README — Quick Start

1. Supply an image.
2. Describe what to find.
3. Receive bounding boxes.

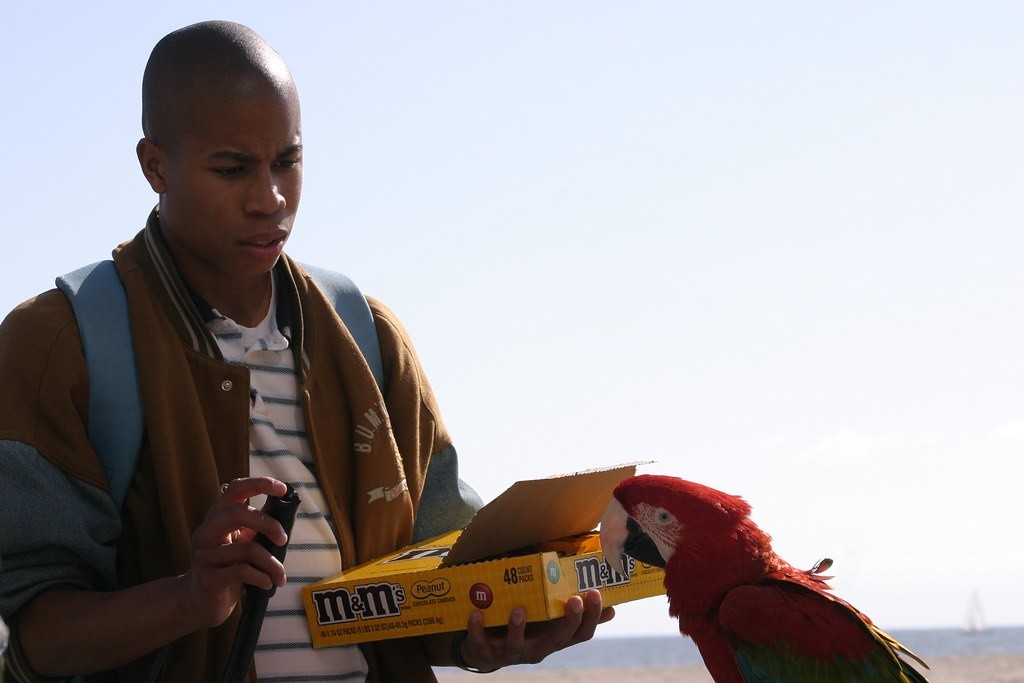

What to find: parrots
[599,474,932,683]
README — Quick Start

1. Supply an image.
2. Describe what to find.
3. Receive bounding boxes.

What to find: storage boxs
[299,458,667,651]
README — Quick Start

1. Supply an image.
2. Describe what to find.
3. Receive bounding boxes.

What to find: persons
[0,19,616,683]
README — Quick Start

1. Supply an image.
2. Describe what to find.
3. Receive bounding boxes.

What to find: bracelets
[450,630,502,673]
